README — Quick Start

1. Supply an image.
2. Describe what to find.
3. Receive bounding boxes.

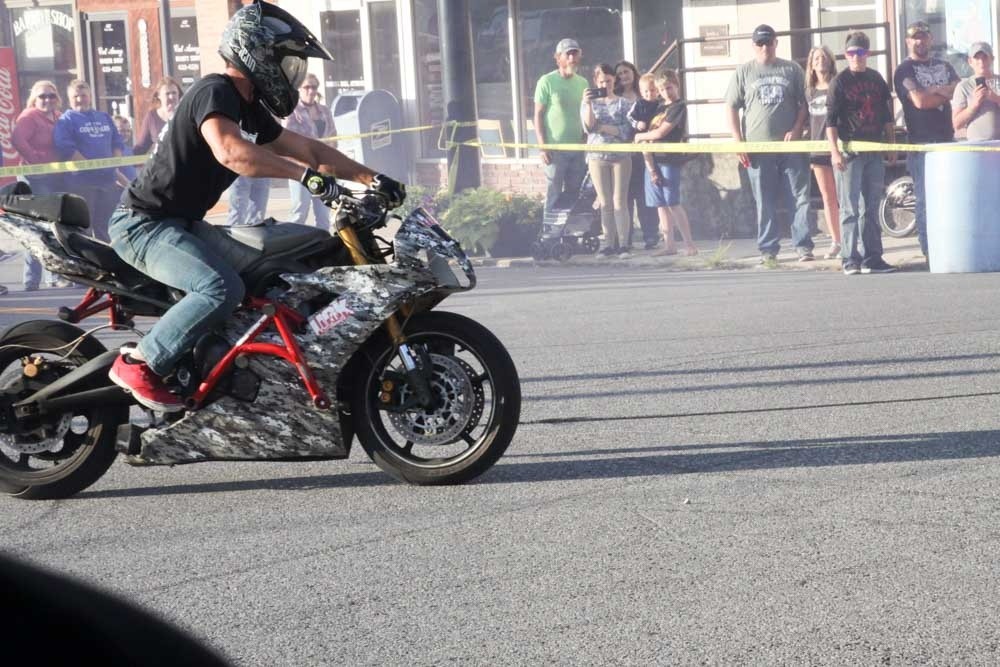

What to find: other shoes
[823,242,842,258]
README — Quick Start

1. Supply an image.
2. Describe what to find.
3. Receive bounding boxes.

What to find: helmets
[217,0,335,120]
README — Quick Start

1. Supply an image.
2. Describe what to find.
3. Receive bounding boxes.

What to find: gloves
[300,167,339,205]
[371,174,407,208]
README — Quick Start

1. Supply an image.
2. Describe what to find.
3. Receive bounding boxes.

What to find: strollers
[532,169,604,262]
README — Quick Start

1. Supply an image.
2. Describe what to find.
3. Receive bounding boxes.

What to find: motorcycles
[0,177,521,499]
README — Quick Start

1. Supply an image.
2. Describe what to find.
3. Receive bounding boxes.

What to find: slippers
[651,247,677,256]
[686,247,699,257]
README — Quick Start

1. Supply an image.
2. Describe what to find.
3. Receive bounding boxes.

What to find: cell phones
[975,77,986,88]
[586,87,607,99]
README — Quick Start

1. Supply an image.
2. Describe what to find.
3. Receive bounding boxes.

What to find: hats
[905,20,930,38]
[556,39,582,55]
[968,40,993,58]
[752,24,776,42]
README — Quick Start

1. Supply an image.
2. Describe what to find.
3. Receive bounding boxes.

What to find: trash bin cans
[332,88,408,184]
[925,140,1000,273]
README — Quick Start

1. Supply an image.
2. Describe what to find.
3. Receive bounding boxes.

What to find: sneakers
[616,247,633,259]
[798,252,816,262]
[842,255,862,275]
[598,247,616,257]
[861,258,899,274]
[108,352,186,413]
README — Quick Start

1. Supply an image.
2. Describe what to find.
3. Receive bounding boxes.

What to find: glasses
[754,40,774,46]
[846,48,866,57]
[36,92,58,99]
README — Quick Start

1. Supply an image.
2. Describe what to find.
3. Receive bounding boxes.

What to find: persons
[951,42,1000,141]
[894,21,962,271]
[724,24,815,261]
[804,46,842,260]
[108,0,407,412]
[533,38,589,242]
[826,31,897,276]
[579,61,699,259]
[0,76,183,291]
[281,73,337,232]
[226,175,269,226]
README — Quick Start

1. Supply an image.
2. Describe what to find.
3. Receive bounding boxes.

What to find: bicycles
[877,176,918,237]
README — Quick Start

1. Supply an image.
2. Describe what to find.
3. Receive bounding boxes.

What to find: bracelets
[967,106,978,115]
